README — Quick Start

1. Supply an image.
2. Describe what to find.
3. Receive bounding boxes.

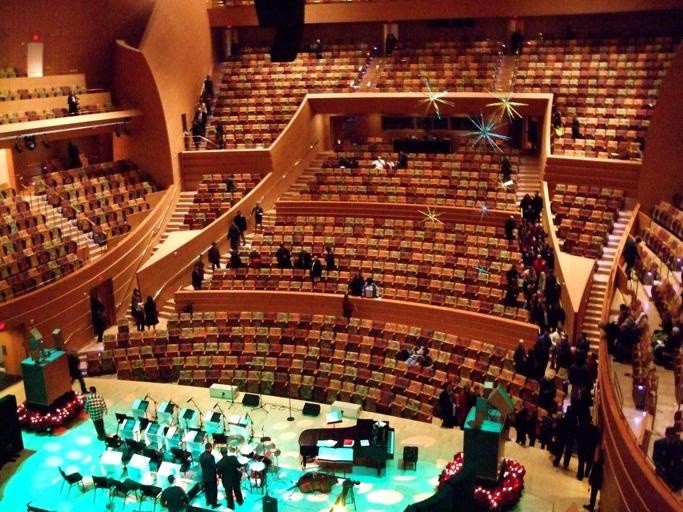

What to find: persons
[68,90,79,112]
[161,475,189,512]
[371,150,411,169]
[227,174,235,192]
[192,201,334,290]
[84,387,107,440]
[132,287,159,331]
[505,191,567,331]
[190,75,225,150]
[623,236,642,280]
[440,382,489,429]
[652,426,682,494]
[405,342,434,369]
[339,156,358,168]
[600,303,642,365]
[651,312,682,365]
[386,33,397,57]
[571,116,580,138]
[584,450,606,511]
[343,272,379,324]
[68,141,81,166]
[68,348,90,394]
[553,107,562,128]
[199,443,243,509]
[90,294,107,342]
[502,158,511,183]
[513,328,601,481]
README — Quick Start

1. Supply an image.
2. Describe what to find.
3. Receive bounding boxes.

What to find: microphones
[144,394,148,400]
[213,402,218,409]
[187,398,192,403]
[245,413,248,419]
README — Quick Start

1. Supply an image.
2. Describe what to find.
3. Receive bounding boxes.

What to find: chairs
[0,35,683,422]
[56,413,250,512]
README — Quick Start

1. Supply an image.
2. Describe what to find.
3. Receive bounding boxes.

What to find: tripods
[226,375,242,409]
[251,375,269,414]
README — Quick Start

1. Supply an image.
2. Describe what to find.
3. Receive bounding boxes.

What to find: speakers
[302,403,320,416]
[210,383,239,399]
[242,394,259,407]
[331,400,363,418]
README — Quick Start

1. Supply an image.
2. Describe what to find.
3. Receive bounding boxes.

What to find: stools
[403,447,418,471]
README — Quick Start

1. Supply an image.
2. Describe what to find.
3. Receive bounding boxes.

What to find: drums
[240,445,254,457]
[245,460,266,488]
[236,455,249,472]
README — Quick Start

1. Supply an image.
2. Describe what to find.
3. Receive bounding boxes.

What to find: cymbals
[256,441,275,456]
[227,435,244,447]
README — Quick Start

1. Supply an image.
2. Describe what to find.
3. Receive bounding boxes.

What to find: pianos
[299,419,395,477]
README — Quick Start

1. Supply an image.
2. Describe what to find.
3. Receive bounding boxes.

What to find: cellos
[296,472,360,493]
